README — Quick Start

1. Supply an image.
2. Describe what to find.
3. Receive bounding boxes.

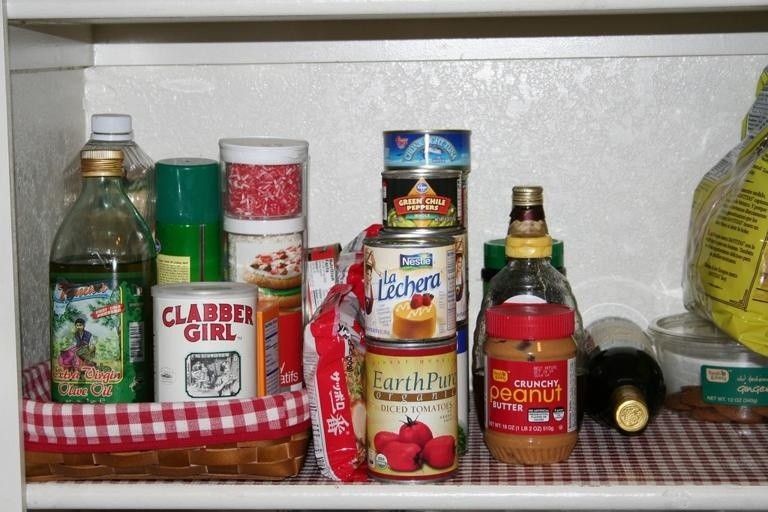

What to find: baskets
[23,357,310,481]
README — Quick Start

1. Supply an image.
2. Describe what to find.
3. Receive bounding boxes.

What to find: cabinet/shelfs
[0,0,768,511]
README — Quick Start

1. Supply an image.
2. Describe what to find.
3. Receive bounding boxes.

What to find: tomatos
[373,414,456,472]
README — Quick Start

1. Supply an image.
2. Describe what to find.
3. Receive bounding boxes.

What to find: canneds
[151,284,260,401]
[483,302,579,465]
[382,128,472,172]
[380,168,464,229]
[362,235,458,343]
[455,333,470,457]
[360,334,458,483]
[376,227,470,331]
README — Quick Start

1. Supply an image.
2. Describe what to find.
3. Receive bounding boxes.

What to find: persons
[61,317,96,371]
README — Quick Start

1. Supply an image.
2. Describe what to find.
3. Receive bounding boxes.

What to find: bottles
[218,137,310,315]
[59,113,158,247]
[152,156,223,284]
[471,185,587,436]
[583,315,668,435]
[48,148,160,406]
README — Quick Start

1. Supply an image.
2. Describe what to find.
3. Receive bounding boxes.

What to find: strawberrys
[411,292,434,308]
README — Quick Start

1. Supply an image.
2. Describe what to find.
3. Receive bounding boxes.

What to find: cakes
[393,301,438,339]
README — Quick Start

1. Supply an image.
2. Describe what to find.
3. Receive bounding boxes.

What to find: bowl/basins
[647,311,768,424]
